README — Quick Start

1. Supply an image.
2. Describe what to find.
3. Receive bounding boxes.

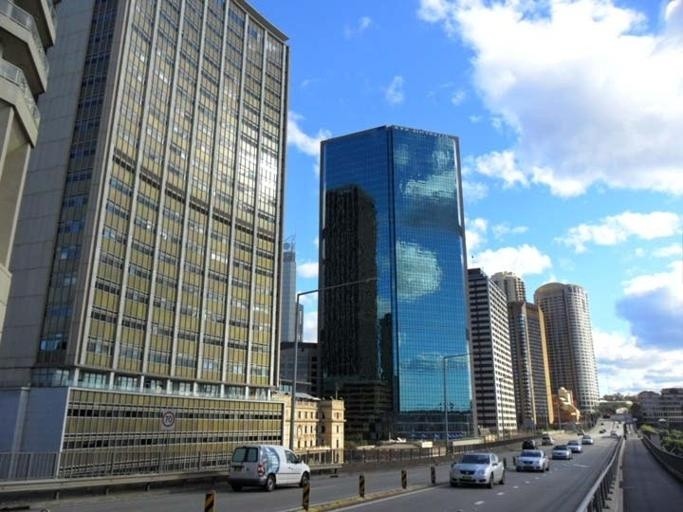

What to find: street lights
[283,274,382,453]
[440,350,484,456]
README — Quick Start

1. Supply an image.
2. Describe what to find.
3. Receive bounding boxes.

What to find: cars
[514,428,595,474]
[448,451,505,490]
[227,444,311,492]
